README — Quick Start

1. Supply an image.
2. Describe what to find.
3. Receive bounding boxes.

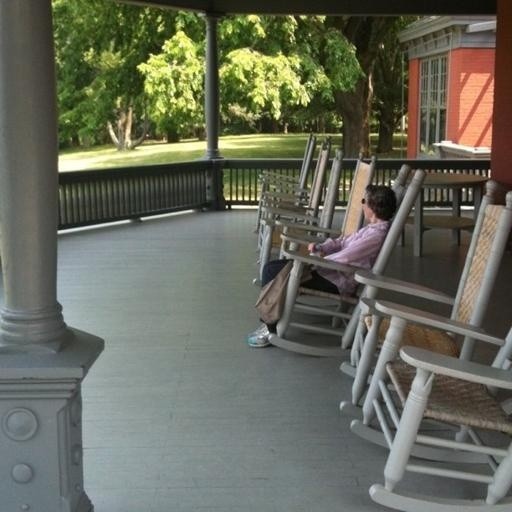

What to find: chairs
[348,302,510,510]
[339,178,510,432]
[255,133,426,357]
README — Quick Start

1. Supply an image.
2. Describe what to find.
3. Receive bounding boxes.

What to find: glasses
[361,198,368,204]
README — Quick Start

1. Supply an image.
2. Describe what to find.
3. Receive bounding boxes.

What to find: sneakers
[247,322,272,347]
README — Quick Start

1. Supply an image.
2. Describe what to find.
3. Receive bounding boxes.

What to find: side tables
[394,173,490,257]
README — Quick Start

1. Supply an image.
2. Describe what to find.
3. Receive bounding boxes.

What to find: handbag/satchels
[255,262,315,326]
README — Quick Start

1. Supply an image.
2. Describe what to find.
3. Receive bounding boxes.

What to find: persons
[248,184,398,349]
